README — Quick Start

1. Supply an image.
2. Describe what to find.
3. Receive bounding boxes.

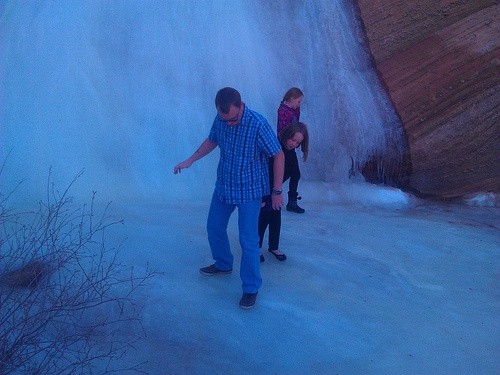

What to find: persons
[173,87,286,309]
[276,87,306,213]
[258,121,309,262]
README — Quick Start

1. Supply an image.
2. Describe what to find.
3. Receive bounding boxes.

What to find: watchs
[272,187,282,195]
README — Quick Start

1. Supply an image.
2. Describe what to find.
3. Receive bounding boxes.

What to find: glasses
[216,111,239,123]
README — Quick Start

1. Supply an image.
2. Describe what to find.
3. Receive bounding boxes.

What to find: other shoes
[268,248,287,262]
[258,253,265,262]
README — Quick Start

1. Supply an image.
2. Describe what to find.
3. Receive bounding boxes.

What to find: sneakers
[199,262,233,276]
[239,290,259,309]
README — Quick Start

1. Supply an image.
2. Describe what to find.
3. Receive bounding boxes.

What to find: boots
[286,191,306,214]
